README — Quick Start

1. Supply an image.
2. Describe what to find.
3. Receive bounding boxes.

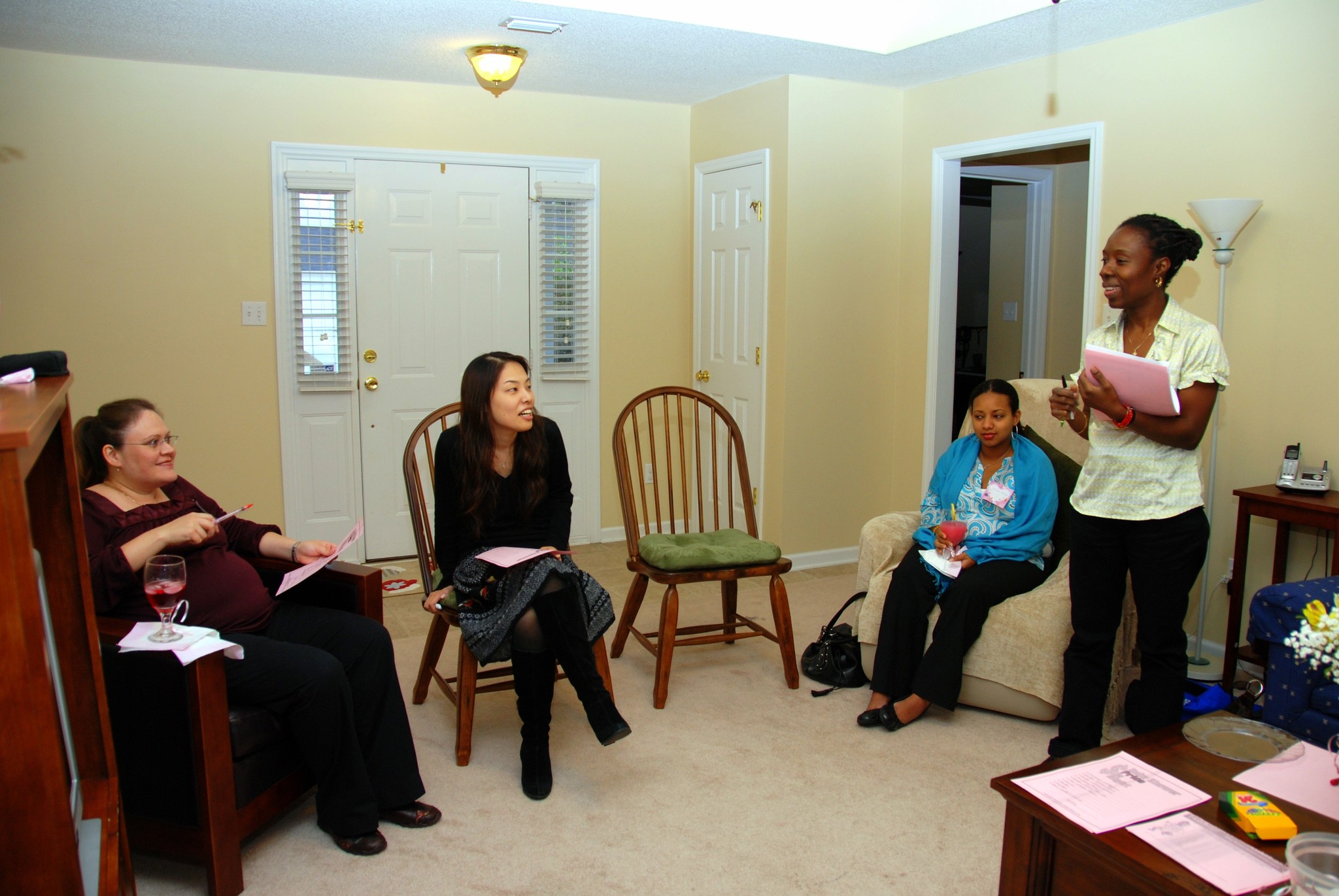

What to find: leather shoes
[857,697,893,725]
[317,820,387,855]
[880,694,931,731]
[373,800,441,828]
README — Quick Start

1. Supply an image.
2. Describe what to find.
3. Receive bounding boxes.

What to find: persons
[73,399,441,856]
[422,351,632,801]
[1042,214,1230,764]
[858,379,1058,731]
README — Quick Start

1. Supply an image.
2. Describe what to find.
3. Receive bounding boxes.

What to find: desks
[1222,483,1339,699]
[990,710,1339,896]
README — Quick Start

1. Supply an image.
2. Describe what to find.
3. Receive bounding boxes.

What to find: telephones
[1276,444,1331,495]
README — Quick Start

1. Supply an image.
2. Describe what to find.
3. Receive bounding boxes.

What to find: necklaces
[493,451,512,469]
[103,482,157,506]
[1125,326,1154,356]
[980,446,1011,469]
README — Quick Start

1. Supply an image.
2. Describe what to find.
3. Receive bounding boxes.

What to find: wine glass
[144,555,188,643]
[941,509,967,562]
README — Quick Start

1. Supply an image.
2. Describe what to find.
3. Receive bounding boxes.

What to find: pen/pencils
[212,504,254,525]
[1060,417,1065,428]
[1061,374,1075,420]
[435,603,442,610]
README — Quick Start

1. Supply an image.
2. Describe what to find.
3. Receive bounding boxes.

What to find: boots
[533,582,633,746]
[512,641,555,798]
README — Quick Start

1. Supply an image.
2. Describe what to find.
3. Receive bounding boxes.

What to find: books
[1083,343,1181,421]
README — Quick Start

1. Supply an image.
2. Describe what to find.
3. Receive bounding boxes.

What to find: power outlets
[1227,557,1234,576]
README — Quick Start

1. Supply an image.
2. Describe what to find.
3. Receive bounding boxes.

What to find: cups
[1285,831,1339,896]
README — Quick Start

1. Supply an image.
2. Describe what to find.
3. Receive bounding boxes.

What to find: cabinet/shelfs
[0,375,135,896]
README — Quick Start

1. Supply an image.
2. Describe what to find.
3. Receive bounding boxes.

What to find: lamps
[466,44,528,87]
[1188,197,1264,682]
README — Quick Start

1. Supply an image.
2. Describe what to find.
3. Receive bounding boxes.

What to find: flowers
[1283,592,1339,684]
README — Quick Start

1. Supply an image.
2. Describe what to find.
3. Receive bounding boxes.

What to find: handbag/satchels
[1125,673,1266,734]
[800,591,870,698]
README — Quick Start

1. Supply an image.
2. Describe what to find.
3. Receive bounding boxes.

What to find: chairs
[608,385,800,712]
[69,414,385,896]
[402,401,620,766]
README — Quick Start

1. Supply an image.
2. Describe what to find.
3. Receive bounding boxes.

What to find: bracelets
[933,525,941,533]
[292,541,301,563]
[1111,404,1136,428]
[1071,411,1087,434]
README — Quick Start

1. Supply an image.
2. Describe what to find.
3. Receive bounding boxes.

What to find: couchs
[1246,574,1339,754]
[853,378,1139,719]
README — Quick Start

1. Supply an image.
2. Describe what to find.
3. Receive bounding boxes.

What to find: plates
[1182,716,1305,763]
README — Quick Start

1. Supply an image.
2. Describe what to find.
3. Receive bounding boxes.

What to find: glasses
[113,435,178,452]
